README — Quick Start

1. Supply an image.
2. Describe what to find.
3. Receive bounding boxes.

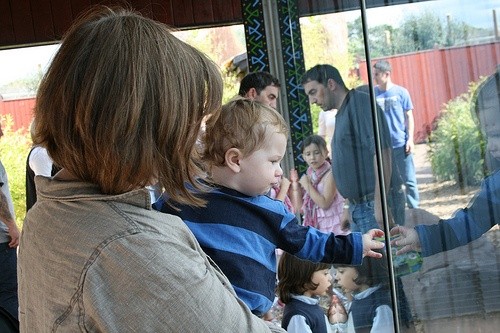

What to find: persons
[238,72,281,108]
[303,64,391,241]
[373,60,419,210]
[18,9,287,332]
[293,136,344,235]
[333,240,395,333]
[391,72,500,256]
[26,120,64,215]
[152,99,385,332]
[278,252,341,333]
[0,125,21,333]
